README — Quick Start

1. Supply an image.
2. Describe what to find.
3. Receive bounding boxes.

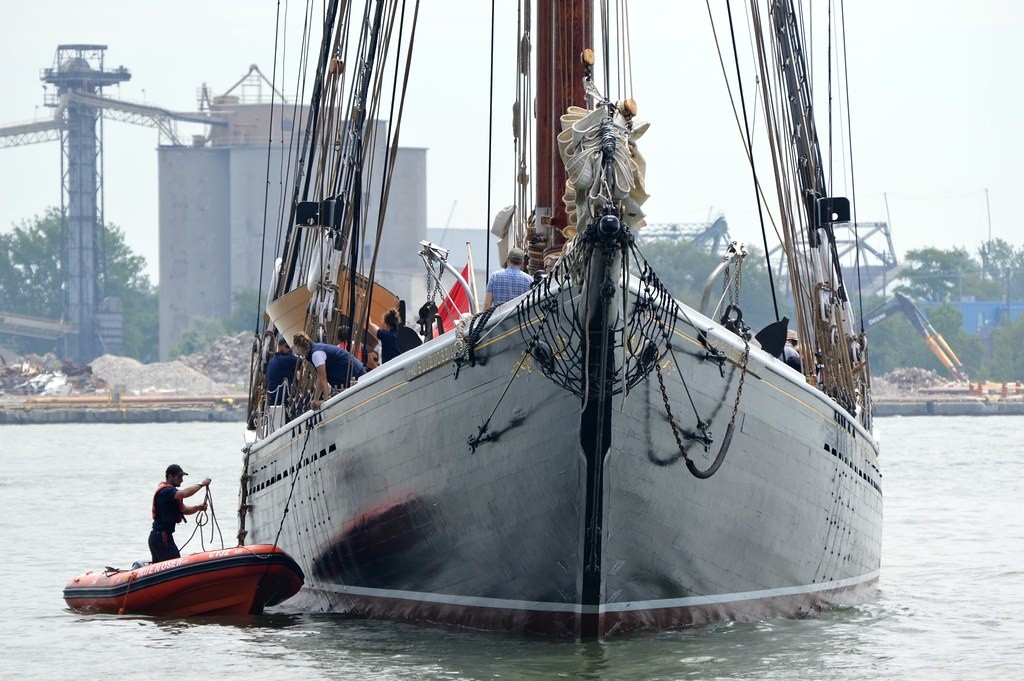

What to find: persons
[294,331,367,410]
[337,325,378,370]
[483,247,535,313]
[777,329,800,364]
[148,464,212,563]
[267,338,303,408]
[357,288,404,364]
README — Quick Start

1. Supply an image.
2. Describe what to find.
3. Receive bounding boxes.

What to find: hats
[165,463,189,476]
[509,248,524,262]
[786,329,799,341]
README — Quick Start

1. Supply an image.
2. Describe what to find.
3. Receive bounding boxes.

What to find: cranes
[0,43,234,369]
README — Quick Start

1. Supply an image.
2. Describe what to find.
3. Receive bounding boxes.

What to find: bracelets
[198,482,203,487]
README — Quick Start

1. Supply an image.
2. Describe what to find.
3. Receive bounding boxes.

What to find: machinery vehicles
[853,290,969,384]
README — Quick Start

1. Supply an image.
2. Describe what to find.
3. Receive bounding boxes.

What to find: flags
[432,262,469,340]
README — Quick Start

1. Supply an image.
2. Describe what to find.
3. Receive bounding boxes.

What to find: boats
[62,537,306,622]
[229,0,882,645]
